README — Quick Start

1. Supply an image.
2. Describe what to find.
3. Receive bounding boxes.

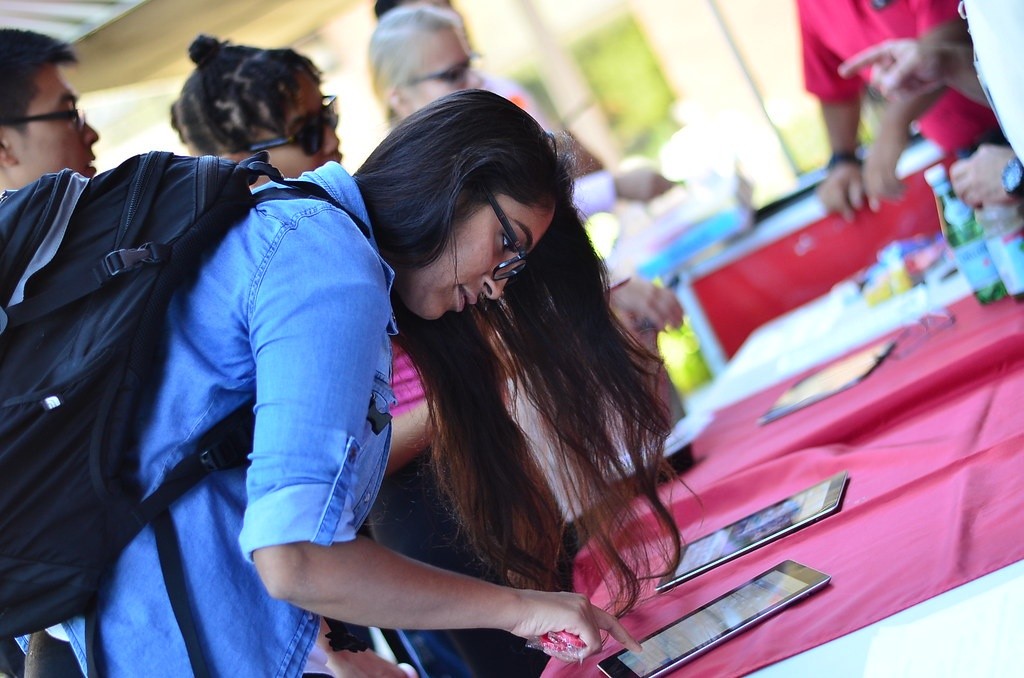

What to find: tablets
[597,560,832,678]
[759,341,896,427]
[655,470,848,592]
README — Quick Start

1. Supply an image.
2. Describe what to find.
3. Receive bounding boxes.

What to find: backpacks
[0,149,396,678]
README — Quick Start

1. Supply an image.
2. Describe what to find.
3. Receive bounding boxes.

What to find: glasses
[6,106,87,133]
[480,183,529,281]
[413,47,480,88]
[250,94,338,156]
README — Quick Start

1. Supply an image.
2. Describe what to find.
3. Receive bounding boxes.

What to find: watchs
[1001,157,1024,197]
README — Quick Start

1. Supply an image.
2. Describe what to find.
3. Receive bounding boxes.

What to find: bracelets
[827,152,863,169]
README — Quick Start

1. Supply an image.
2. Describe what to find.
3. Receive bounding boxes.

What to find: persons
[0,29,98,194]
[837,0,1024,206]
[797,0,1009,219]
[170,36,576,678]
[366,0,684,524]
[13,88,704,660]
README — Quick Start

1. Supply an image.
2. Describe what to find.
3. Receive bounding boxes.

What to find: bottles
[956,150,1023,303]
[924,162,1008,305]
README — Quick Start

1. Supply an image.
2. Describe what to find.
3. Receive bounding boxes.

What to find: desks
[531,264,1024,678]
